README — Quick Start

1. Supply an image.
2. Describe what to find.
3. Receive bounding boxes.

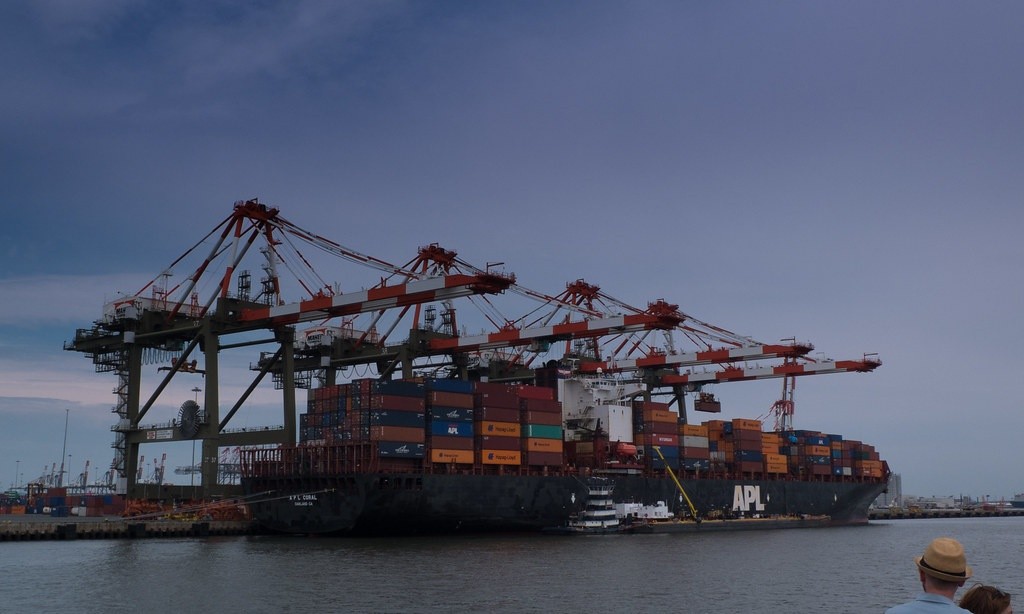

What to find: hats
[914,536,971,582]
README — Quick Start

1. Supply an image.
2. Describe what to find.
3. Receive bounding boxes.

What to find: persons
[885,537,975,614]
[959,583,1012,614]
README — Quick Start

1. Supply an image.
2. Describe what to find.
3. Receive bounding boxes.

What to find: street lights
[192,386,202,486]
[59,408,69,487]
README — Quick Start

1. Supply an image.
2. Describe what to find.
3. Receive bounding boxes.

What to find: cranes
[62,197,516,484]
[470,279,815,377]
[249,242,685,390]
[579,299,882,391]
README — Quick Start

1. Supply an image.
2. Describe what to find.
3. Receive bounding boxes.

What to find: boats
[565,485,619,533]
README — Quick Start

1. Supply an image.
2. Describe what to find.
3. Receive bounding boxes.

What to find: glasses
[992,586,1006,598]
